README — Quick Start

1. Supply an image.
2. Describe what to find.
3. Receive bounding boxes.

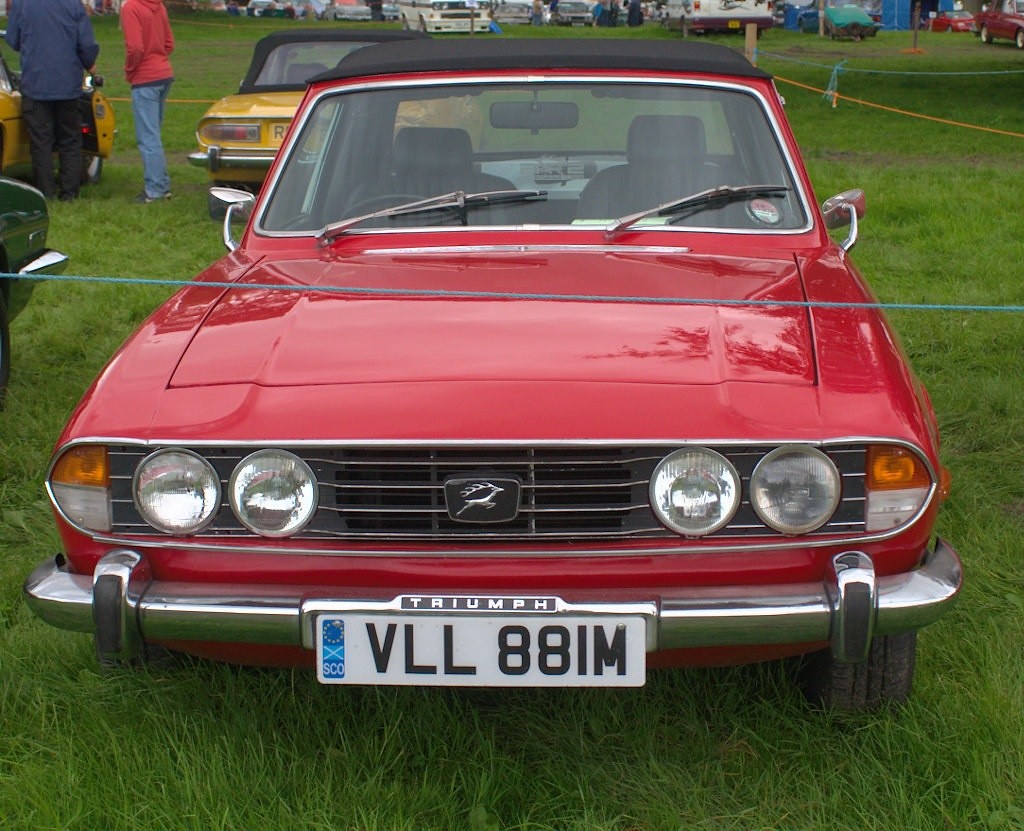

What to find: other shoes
[135,190,171,203]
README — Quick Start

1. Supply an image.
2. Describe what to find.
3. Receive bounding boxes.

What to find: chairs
[285,63,330,84]
[343,126,525,226]
[571,114,750,224]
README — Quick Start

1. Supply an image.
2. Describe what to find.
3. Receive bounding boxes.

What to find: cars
[0,175,70,392]
[22,31,964,722]
[84,0,785,40]
[186,25,487,226]
[925,10,978,33]
[1,31,116,187]
[974,0,1024,50]
[797,0,886,37]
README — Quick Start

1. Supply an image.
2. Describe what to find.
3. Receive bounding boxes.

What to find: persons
[6,0,100,201]
[120,0,176,204]
[222,0,668,28]
